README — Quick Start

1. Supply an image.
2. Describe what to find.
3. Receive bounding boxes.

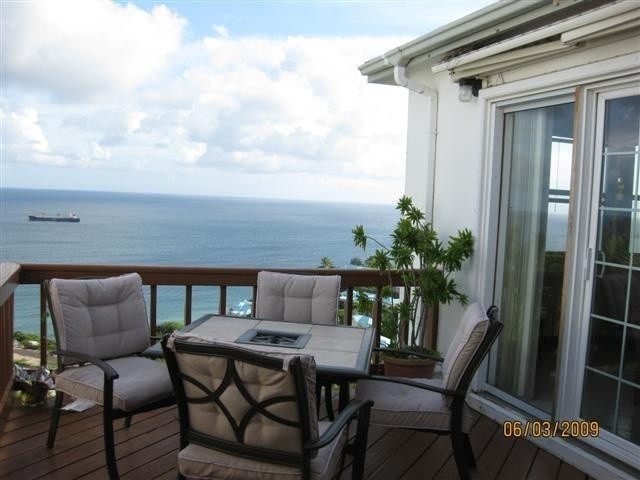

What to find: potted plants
[351,193,474,378]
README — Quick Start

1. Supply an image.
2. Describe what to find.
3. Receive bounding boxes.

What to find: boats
[29,208,80,222]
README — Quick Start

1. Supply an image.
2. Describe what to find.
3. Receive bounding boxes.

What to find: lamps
[457,77,482,104]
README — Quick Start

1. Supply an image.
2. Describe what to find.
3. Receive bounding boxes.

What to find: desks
[141,313,377,479]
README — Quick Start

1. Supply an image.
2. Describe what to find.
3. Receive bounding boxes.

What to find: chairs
[160,330,375,480]
[253,269,342,423]
[598,273,640,375]
[344,302,504,479]
[42,270,176,480]
